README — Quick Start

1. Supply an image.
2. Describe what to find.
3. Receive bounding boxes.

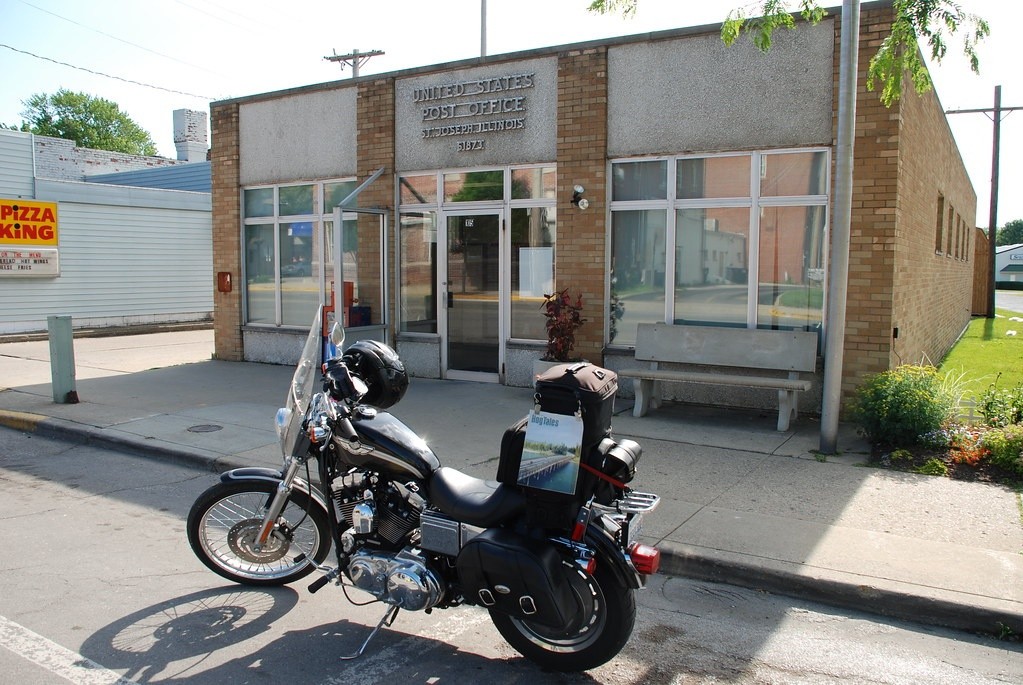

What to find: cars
[280,262,310,277]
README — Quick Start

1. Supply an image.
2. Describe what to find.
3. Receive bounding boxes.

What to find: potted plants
[533,289,589,390]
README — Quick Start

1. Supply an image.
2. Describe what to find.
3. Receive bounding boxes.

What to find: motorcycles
[186,323,662,673]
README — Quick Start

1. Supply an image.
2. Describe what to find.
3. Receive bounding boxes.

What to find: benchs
[617,321,819,432]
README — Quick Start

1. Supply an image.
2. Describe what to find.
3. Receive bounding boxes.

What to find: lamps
[569,185,588,210]
